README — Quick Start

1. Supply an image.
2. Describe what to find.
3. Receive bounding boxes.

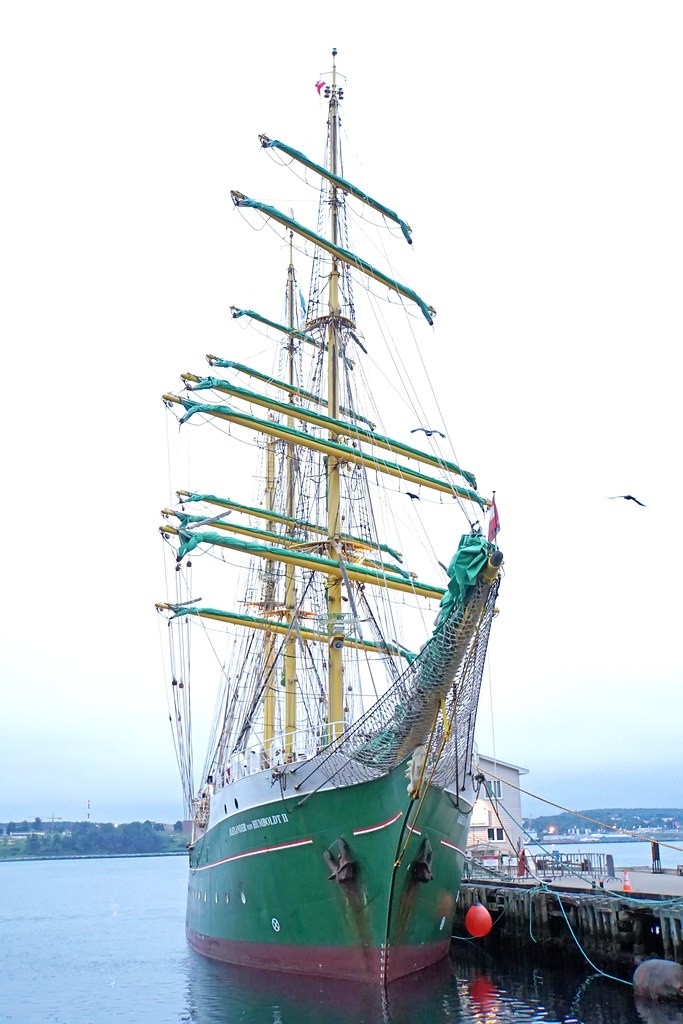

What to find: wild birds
[410,427,447,439]
[607,494,647,509]
[405,492,420,501]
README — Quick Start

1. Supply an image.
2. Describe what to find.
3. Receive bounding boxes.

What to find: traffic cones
[623,871,632,892]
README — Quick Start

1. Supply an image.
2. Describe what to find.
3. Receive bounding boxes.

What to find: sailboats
[121,44,504,1001]
